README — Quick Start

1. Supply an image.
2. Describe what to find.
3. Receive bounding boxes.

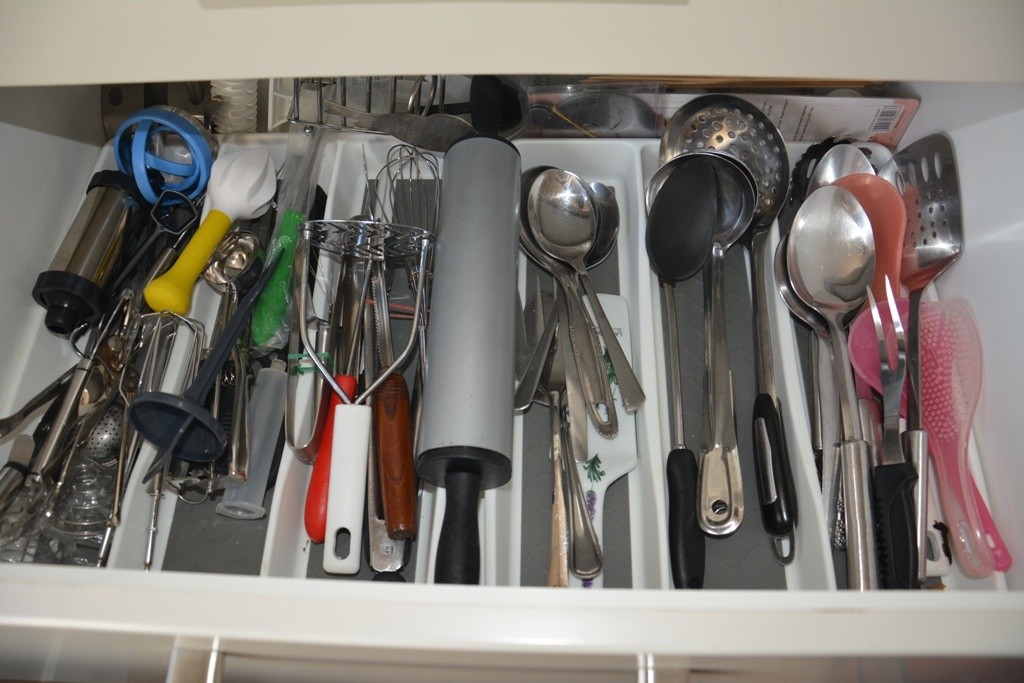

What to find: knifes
[317,216,369,402]
[566,293,637,587]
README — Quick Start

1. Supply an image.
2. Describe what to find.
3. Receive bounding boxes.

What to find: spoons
[514,163,646,588]
[770,138,907,590]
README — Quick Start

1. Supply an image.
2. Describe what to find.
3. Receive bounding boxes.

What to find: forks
[864,273,916,589]
[514,274,604,578]
[194,231,238,283]
